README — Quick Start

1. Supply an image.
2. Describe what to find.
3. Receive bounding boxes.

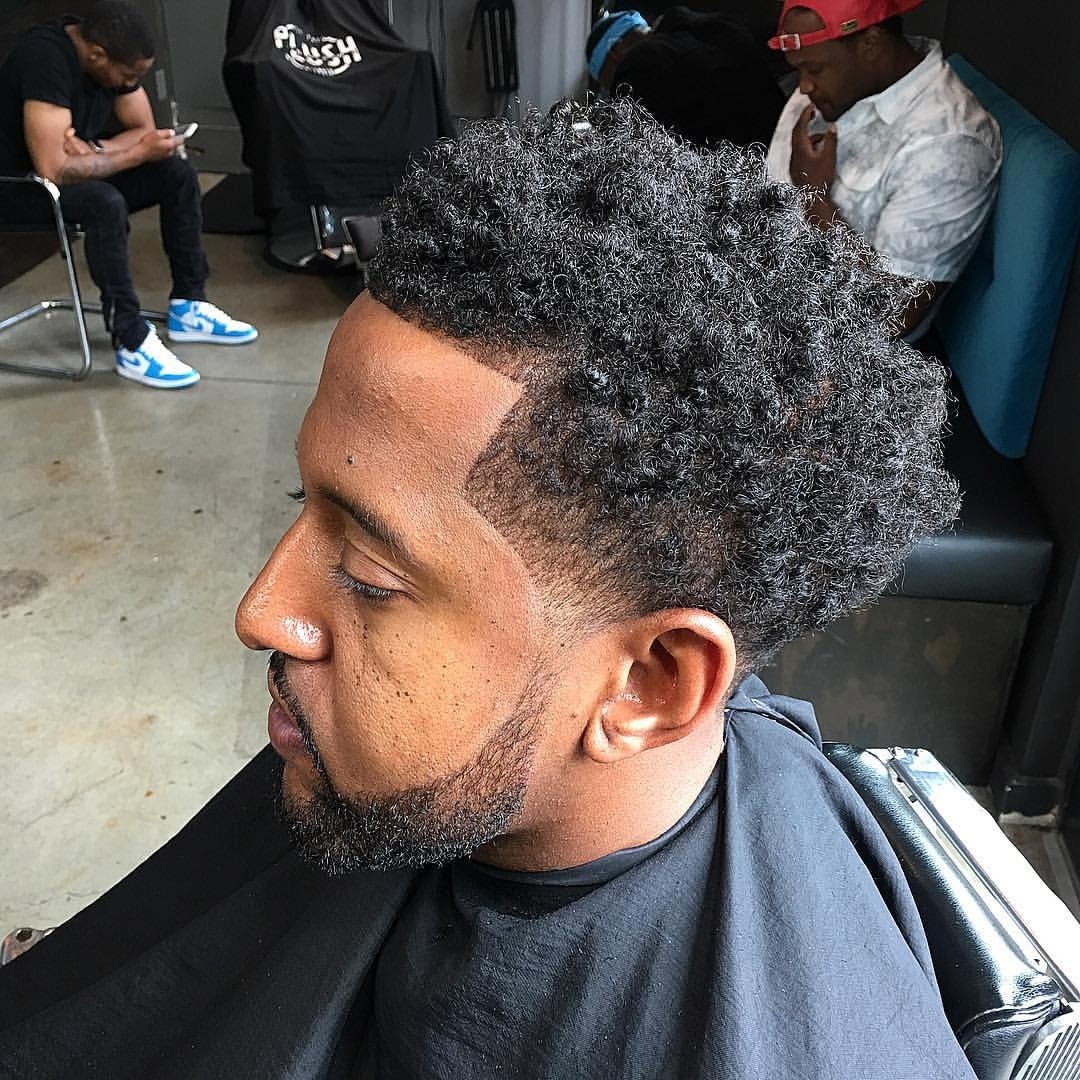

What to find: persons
[759,1,1006,348]
[0,90,972,1078]
[2,9,260,390]
[584,10,785,154]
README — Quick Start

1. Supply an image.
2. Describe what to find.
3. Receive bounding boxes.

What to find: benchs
[754,47,1080,785]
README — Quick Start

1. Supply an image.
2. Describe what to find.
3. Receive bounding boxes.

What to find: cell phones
[174,122,199,139]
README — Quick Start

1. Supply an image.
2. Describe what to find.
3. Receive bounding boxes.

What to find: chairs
[225,48,447,276]
[810,739,1080,1079]
[0,175,171,381]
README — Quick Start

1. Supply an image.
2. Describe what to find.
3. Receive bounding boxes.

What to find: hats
[768,0,924,51]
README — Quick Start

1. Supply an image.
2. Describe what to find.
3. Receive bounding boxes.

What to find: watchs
[88,138,104,154]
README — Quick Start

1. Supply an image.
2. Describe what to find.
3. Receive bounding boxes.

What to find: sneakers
[167,298,259,344]
[115,320,200,389]
